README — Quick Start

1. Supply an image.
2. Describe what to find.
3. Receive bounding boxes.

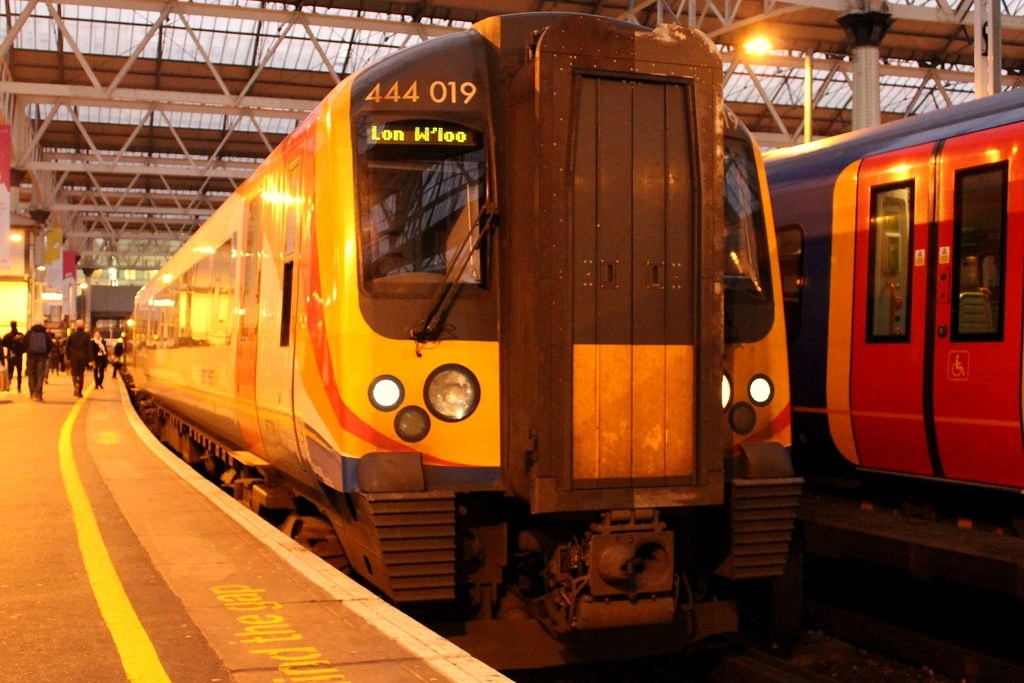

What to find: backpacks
[11,335,25,354]
[27,331,47,354]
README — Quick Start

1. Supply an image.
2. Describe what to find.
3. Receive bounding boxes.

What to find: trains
[747,84,1024,599]
[118,11,806,639]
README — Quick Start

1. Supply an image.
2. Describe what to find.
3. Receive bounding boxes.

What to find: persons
[0,320,124,402]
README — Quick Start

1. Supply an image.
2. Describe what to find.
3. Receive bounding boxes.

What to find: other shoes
[77,390,83,397]
[74,391,77,396]
